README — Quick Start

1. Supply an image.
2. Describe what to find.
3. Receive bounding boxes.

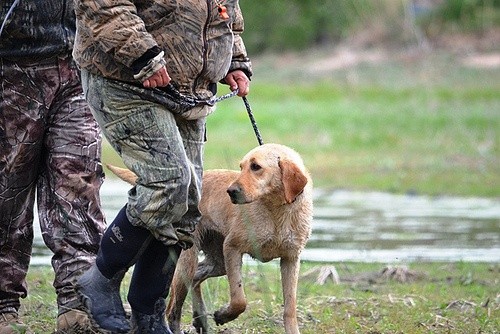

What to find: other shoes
[57,304,112,334]
[0,311,34,334]
[74,261,130,332]
[131,306,172,334]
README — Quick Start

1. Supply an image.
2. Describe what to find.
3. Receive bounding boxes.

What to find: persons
[73,0,253,334]
[0,0,108,334]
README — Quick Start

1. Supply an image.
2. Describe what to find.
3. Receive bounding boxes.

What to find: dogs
[103,143,315,333]
